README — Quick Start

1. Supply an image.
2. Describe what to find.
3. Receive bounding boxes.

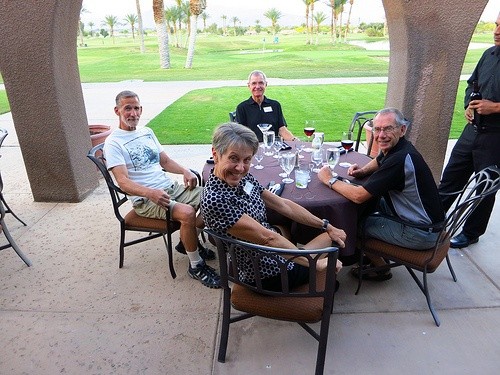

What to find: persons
[102,91,221,289]
[236,71,300,141]
[435,11,500,249]
[200,122,347,295]
[318,107,445,281]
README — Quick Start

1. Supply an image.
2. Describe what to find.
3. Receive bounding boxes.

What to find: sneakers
[174,239,216,261]
[187,260,222,288]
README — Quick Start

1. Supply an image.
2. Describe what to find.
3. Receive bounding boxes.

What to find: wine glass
[263,131,275,156]
[312,132,325,155]
[325,148,340,178]
[272,136,283,159]
[311,145,323,173]
[304,120,316,153]
[281,153,296,184]
[257,123,272,148]
[278,151,292,178]
[253,143,265,170]
[339,131,354,168]
[294,136,305,158]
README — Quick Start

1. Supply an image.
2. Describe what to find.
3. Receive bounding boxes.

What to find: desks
[197,140,383,289]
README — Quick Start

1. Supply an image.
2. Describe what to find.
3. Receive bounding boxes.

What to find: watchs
[328,178,338,188]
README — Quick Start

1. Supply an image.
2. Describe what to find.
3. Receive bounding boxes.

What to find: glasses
[372,123,403,133]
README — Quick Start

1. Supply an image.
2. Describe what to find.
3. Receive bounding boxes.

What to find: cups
[294,169,310,189]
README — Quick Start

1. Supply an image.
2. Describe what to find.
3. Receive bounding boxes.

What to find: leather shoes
[450,232,479,249]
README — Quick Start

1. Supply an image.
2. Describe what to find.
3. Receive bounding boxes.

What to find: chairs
[200,223,350,375]
[87,142,203,283]
[352,163,500,328]
[226,108,237,126]
[0,126,34,267]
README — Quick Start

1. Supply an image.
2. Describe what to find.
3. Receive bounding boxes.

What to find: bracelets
[322,219,329,231]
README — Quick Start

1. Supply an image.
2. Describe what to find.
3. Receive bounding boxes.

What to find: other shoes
[334,280,339,295]
[350,266,392,281]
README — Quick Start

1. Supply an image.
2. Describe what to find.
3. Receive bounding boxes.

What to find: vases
[88,122,112,173]
[360,118,381,156]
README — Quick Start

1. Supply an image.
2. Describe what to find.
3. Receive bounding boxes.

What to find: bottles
[469,79,488,134]
